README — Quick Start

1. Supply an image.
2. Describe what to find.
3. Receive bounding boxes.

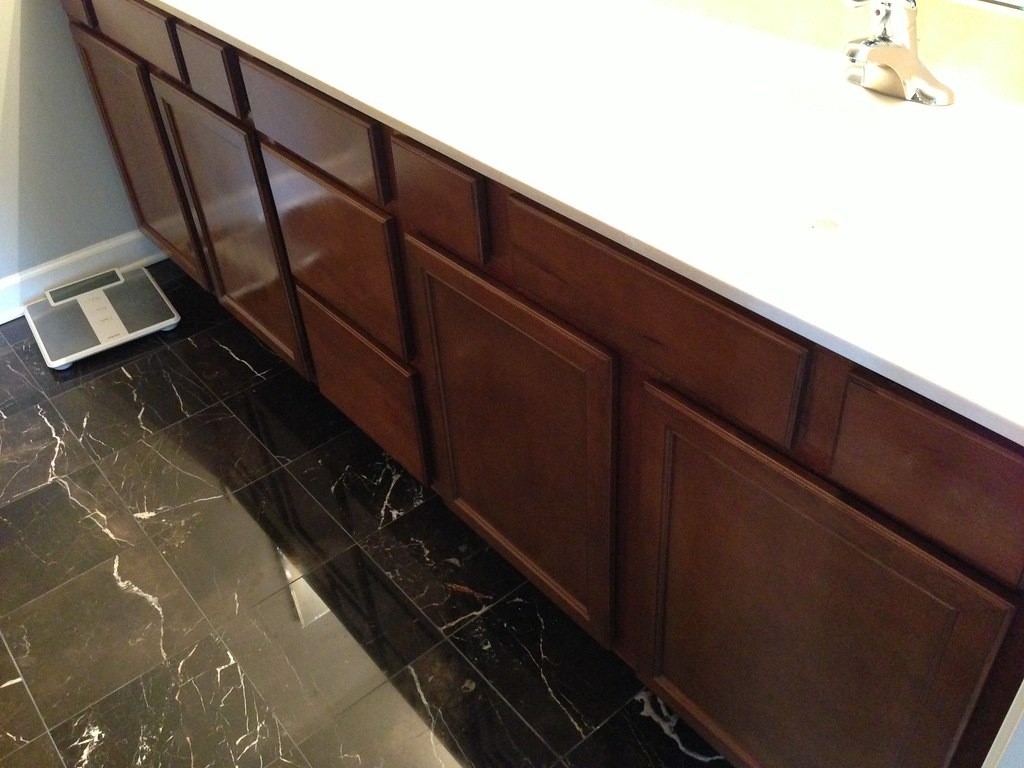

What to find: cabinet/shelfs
[60,2,1024,768]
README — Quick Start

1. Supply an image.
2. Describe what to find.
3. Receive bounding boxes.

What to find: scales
[22,265,182,371]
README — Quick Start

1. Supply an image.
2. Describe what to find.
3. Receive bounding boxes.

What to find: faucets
[847,37,954,106]
